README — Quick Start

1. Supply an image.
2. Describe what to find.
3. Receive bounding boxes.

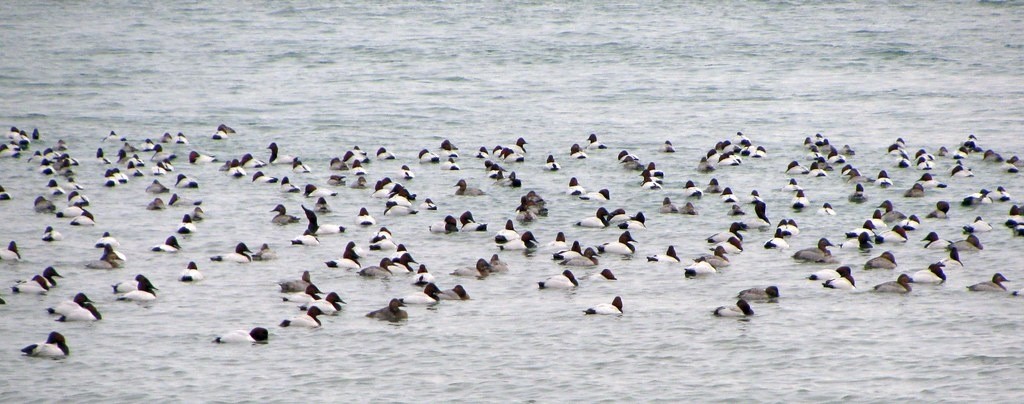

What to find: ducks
[1,124,1024,360]
